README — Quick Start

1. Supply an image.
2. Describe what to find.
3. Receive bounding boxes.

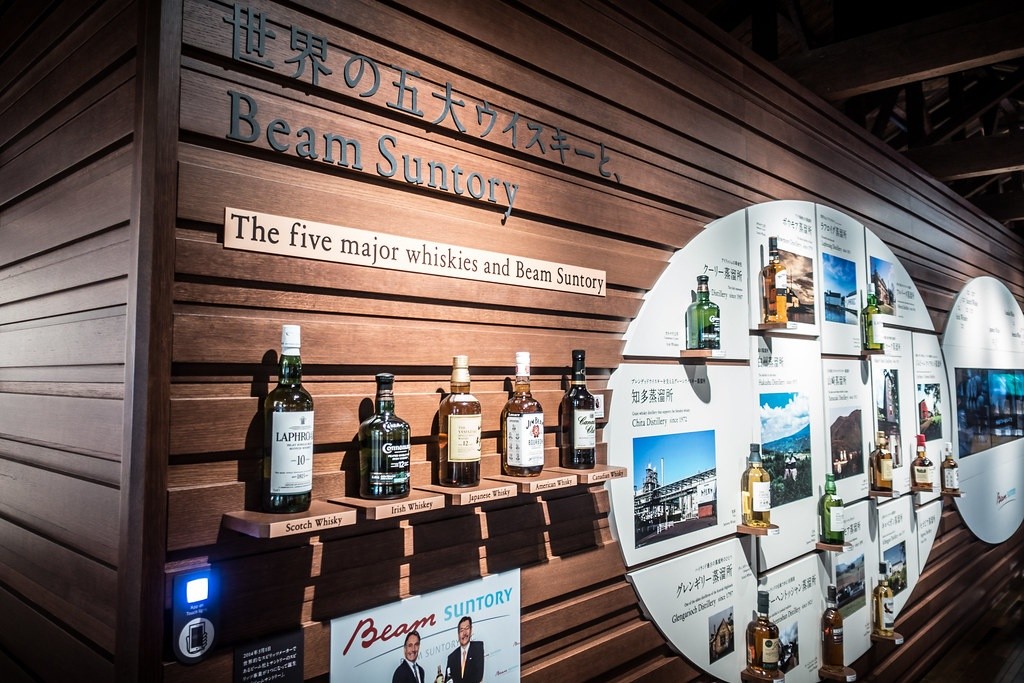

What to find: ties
[461,651,466,679]
[413,665,418,683]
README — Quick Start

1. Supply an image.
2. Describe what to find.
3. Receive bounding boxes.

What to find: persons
[391,631,426,683]
[444,616,485,683]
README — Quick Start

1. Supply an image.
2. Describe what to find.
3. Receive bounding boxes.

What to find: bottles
[741,443,771,527]
[746,591,779,677]
[358,372,411,500]
[438,355,482,488]
[821,586,844,673]
[560,349,596,469]
[501,352,545,477]
[261,324,314,514]
[686,275,721,349]
[990,399,1024,437]
[818,473,845,544]
[861,282,884,350]
[911,434,934,489]
[869,431,893,492]
[872,562,894,637]
[762,236,789,322]
[940,442,959,494]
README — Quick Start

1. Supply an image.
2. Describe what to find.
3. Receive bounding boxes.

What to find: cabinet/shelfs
[679,324,967,683]
